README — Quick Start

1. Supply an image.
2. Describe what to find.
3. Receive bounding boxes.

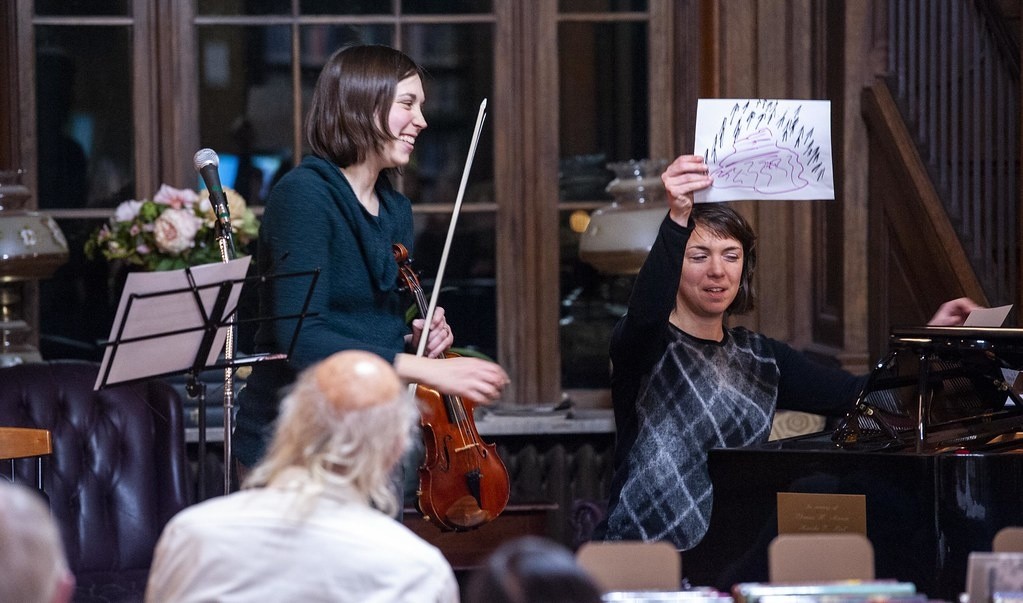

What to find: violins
[392,241,511,534]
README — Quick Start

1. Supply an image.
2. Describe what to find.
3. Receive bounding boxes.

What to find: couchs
[0,359,193,603]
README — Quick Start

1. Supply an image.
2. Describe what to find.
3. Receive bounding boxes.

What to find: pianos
[673,395,1023,603]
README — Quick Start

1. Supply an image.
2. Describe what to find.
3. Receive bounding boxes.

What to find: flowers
[84,182,262,272]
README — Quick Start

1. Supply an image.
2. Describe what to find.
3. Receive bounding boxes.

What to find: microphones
[193,148,233,234]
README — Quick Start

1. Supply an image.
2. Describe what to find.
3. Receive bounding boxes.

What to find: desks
[403,408,615,584]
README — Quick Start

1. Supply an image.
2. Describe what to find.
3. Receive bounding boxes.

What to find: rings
[442,326,450,336]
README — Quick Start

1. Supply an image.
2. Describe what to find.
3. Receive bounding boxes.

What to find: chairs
[768,533,875,585]
[0,427,53,509]
[574,540,681,595]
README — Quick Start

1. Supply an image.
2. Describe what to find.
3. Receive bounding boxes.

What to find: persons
[865,455,1023,603]
[589,153,988,592]
[0,480,78,603]
[231,43,513,523]
[142,348,461,603]
[461,531,608,603]
